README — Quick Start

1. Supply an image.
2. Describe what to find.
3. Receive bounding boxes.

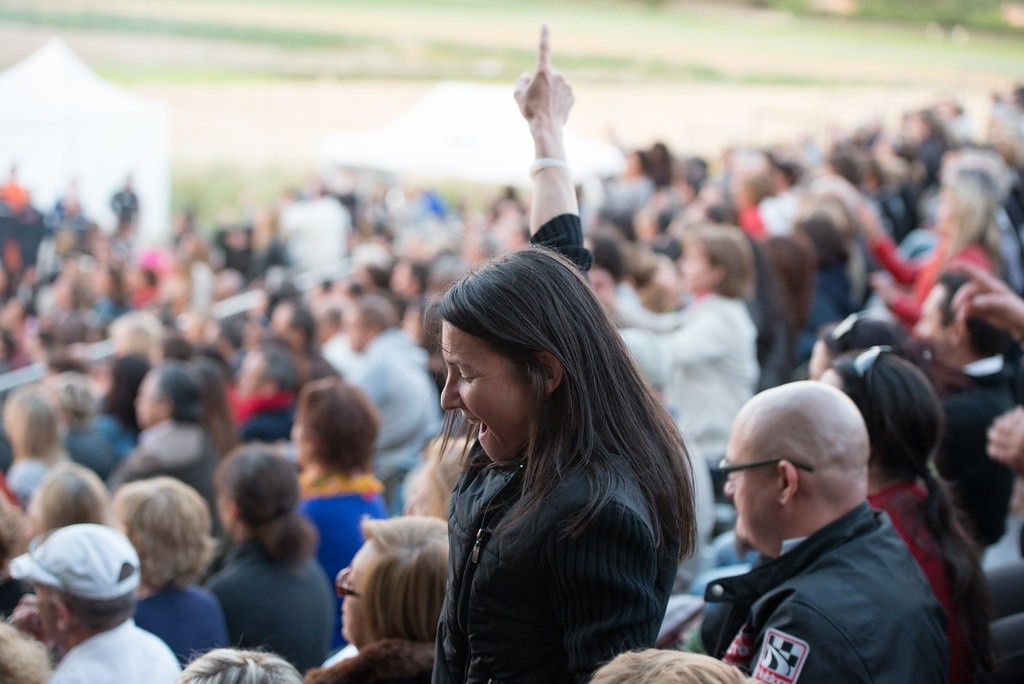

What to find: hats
[9,523,142,600]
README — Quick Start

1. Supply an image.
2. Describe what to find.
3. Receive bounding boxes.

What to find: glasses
[830,310,872,351]
[852,344,904,407]
[336,566,365,603]
[720,458,814,480]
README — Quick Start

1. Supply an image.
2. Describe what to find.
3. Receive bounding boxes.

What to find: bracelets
[529,158,572,178]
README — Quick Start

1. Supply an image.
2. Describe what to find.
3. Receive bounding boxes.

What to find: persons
[429,22,696,683]
[1,87,1024,683]
